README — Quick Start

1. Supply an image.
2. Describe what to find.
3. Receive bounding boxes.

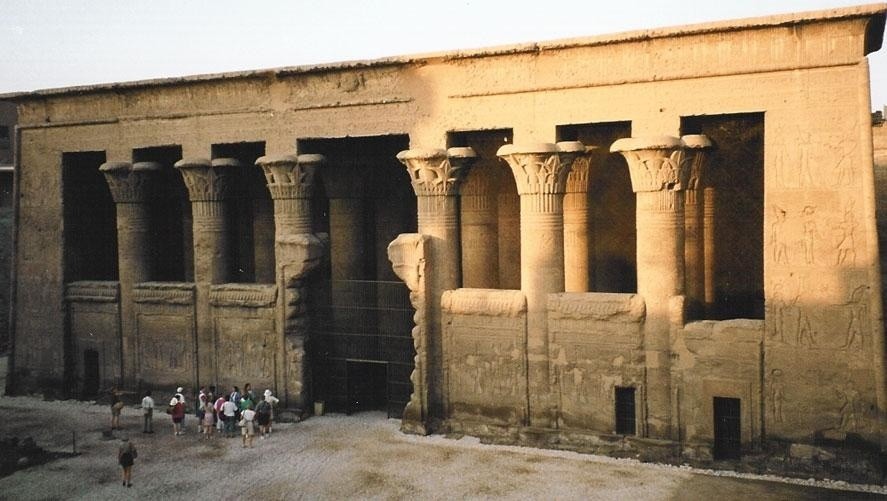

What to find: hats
[170,398,178,405]
[264,390,271,396]
[177,387,182,392]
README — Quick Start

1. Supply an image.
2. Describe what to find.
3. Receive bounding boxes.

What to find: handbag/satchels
[219,412,225,420]
[120,454,134,465]
[238,419,246,427]
[114,402,123,410]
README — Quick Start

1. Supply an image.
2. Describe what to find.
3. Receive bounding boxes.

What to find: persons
[117,434,137,488]
[108,386,125,433]
[141,391,154,435]
[166,382,280,450]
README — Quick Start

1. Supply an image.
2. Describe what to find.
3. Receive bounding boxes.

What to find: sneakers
[175,432,185,435]
[112,427,121,430]
[143,431,153,434]
[123,482,125,485]
[128,484,132,487]
